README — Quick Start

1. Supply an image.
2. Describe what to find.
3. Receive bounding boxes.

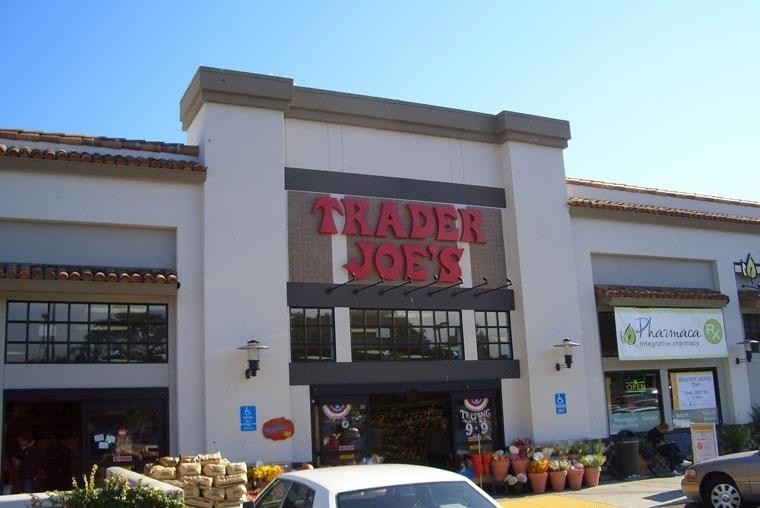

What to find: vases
[492,460,602,493]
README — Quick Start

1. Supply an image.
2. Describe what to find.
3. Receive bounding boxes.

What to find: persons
[647,423,693,475]
[11,433,37,494]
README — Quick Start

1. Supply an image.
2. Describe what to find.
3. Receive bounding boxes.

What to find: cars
[243,464,502,508]
[681,450,759,508]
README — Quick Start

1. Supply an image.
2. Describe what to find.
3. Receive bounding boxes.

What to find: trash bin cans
[617,441,640,478]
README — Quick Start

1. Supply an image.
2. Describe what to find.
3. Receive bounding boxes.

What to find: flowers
[492,437,609,472]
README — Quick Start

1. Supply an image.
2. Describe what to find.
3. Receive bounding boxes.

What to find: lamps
[735,338,759,364]
[237,340,268,380]
[553,338,579,371]
[327,272,513,298]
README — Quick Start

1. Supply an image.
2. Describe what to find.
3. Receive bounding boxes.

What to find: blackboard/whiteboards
[690,423,718,464]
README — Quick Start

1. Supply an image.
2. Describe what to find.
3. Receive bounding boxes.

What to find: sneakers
[680,460,692,466]
[671,470,681,475]
